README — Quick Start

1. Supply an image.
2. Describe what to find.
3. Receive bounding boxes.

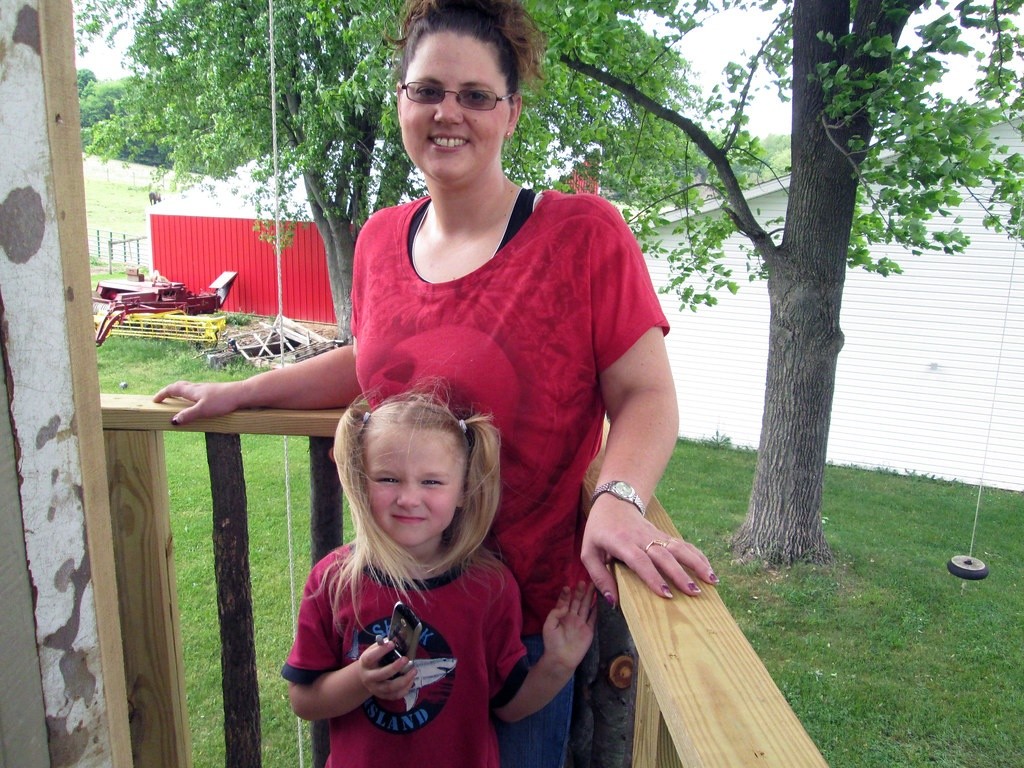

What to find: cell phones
[376,602,423,681]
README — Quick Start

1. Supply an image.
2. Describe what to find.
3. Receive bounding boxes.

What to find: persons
[152,0,719,768]
[282,375,597,768]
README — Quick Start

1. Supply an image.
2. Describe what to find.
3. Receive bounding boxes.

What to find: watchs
[589,480,646,517]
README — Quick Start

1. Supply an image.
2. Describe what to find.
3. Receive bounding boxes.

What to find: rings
[645,539,664,554]
[663,540,670,548]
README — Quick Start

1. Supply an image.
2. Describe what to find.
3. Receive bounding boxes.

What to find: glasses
[400,81,512,111]
